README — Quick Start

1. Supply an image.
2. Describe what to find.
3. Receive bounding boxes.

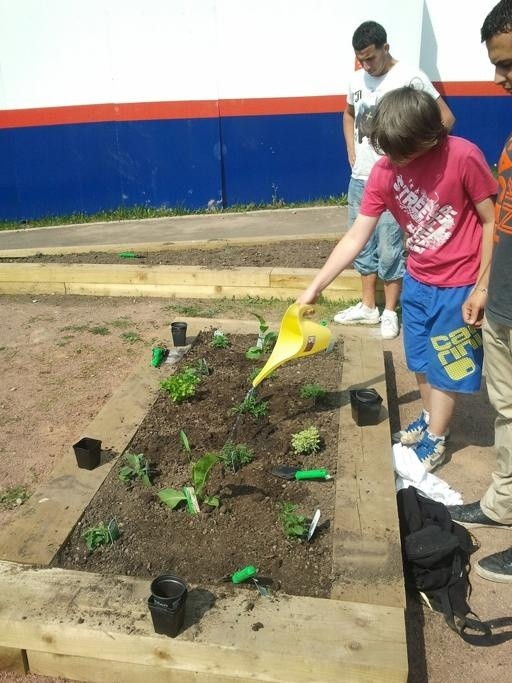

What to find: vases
[350,388,383,427]
[146,575,189,639]
[71,437,103,471]
[170,321,189,346]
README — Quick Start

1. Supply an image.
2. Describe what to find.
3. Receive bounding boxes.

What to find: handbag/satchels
[397,485,472,617]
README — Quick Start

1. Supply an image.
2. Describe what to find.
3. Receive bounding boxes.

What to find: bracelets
[475,286,488,296]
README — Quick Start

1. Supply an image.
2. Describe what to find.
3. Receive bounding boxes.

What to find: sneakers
[473,545,512,585]
[448,500,512,529]
[380,309,401,340]
[414,430,446,472]
[334,302,380,324]
[393,409,452,445]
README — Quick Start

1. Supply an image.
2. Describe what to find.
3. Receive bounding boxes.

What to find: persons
[296,84,500,474]
[334,20,456,340]
[441,0,512,584]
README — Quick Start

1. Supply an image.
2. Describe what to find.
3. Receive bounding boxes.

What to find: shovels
[222,566,258,584]
[268,466,329,482]
